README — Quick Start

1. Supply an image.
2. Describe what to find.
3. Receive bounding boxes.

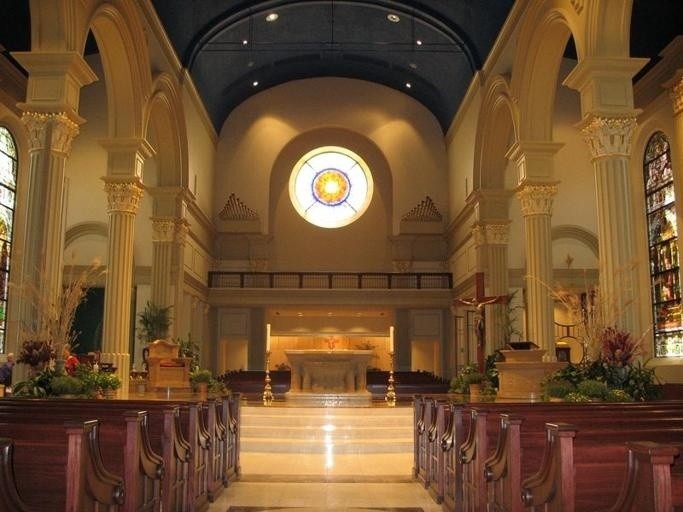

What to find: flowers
[15,339,56,367]
[30,364,43,382]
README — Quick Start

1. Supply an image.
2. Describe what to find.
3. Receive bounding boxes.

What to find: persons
[65,344,79,375]
[0,352,14,386]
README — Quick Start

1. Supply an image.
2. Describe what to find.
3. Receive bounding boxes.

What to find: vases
[55,360,68,380]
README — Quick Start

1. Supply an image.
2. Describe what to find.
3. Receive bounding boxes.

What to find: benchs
[412,393,683,512]
[0,392,241,512]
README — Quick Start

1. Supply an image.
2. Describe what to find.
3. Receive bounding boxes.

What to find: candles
[389,325,394,352]
[266,323,271,352]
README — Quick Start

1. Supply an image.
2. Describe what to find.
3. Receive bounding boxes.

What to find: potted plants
[578,381,607,402]
[191,370,213,394]
[545,384,570,402]
[461,373,487,398]
